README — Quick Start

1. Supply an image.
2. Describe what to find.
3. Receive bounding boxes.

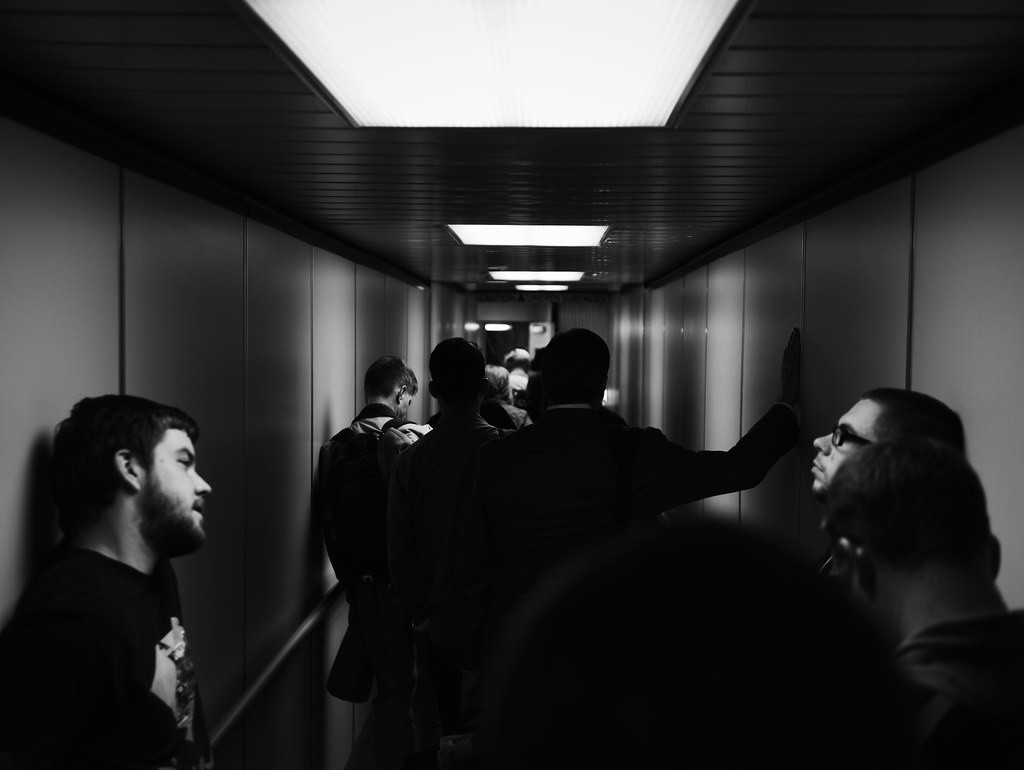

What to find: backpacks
[328,420,408,574]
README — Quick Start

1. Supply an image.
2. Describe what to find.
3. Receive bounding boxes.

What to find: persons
[1,392,225,770]
[316,317,1023,769]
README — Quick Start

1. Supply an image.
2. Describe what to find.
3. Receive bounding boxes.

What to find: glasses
[831,425,872,447]
